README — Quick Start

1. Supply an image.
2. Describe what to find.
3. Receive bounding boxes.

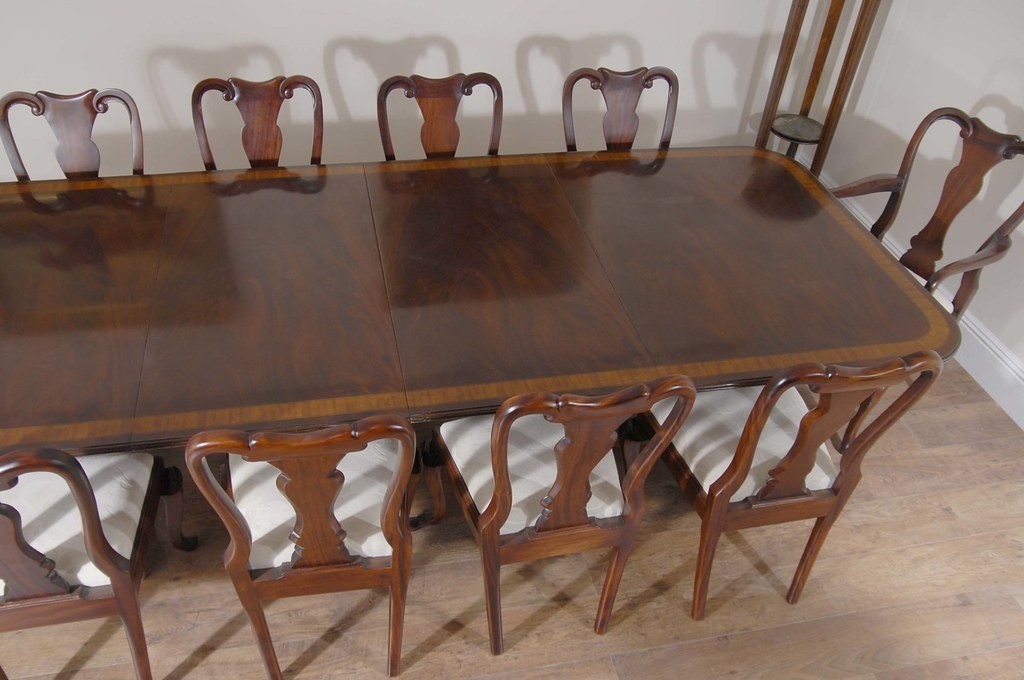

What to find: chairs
[420,376,696,656]
[0,447,199,680]
[831,107,1024,454]
[623,350,942,622]
[184,415,424,680]
[561,67,678,152]
[0,88,143,182]
[191,74,323,171]
[377,72,503,162]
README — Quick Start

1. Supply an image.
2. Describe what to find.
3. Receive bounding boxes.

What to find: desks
[0,146,963,496]
